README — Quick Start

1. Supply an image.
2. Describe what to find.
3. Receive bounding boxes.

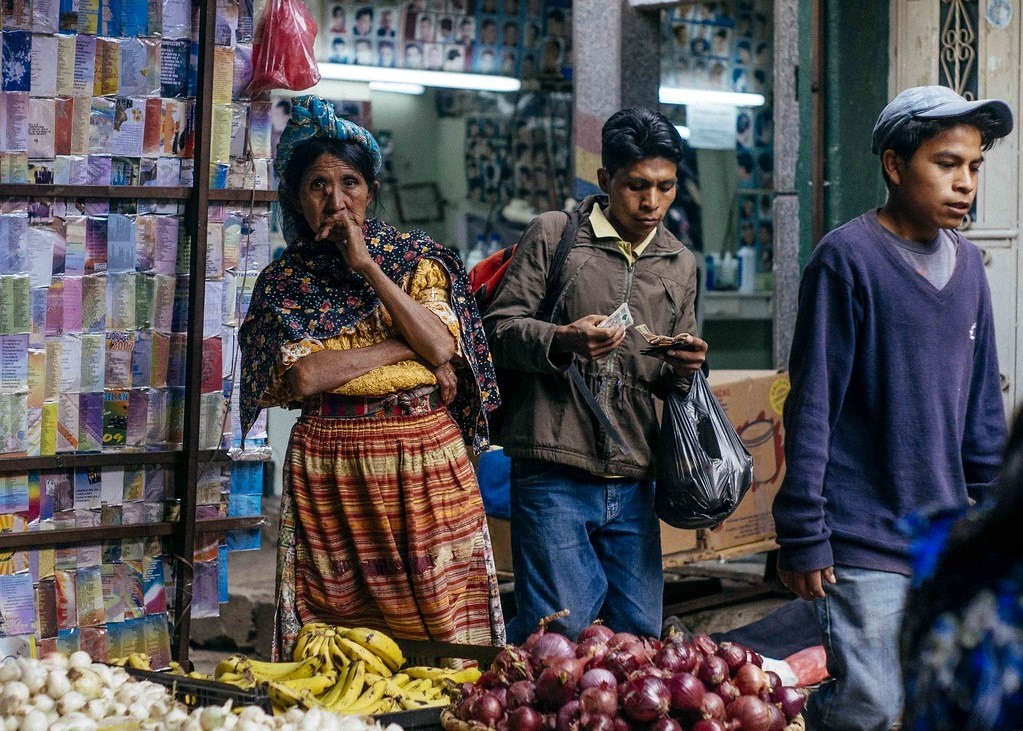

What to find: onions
[447,619,809,731]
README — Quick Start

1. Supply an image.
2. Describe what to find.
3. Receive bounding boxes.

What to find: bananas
[107,620,482,716]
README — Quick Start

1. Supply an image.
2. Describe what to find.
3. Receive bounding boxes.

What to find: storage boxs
[0,637,505,731]
[463,369,795,572]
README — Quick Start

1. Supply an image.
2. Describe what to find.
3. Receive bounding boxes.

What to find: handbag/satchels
[485,90,568,252]
[245,0,321,91]
[654,367,754,531]
[466,209,581,445]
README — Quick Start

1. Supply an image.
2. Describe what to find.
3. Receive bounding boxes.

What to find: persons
[771,85,1014,731]
[237,95,507,663]
[900,400,1023,731]
[479,0,574,78]
[674,3,771,93]
[465,117,551,211]
[736,110,773,271]
[484,108,708,647]
[275,100,291,129]
[34,165,54,184]
[328,1,470,70]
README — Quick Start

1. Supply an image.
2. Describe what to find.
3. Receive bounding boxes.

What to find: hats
[871,86,1014,157]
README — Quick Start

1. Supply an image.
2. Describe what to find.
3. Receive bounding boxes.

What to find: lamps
[659,87,765,107]
[316,62,521,95]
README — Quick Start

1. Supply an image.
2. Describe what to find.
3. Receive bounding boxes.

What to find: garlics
[0,651,407,731]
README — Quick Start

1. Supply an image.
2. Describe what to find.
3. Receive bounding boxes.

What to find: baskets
[93,639,505,731]
